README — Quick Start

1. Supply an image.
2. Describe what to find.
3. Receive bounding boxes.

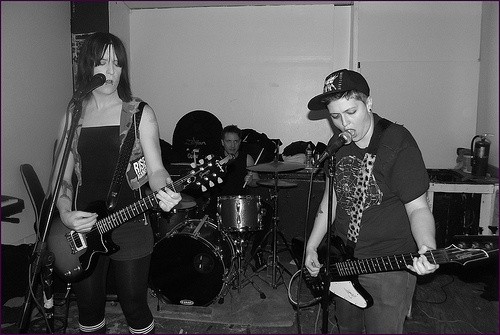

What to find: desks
[424,168,499,238]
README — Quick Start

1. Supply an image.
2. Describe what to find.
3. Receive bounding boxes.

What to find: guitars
[45,152,233,281]
[292,235,499,309]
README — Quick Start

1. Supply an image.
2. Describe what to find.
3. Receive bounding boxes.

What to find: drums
[148,219,236,306]
[217,195,265,233]
[149,192,197,241]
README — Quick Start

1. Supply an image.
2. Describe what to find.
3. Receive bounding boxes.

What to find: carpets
[102,252,300,327]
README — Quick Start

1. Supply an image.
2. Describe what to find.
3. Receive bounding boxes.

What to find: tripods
[217,174,302,310]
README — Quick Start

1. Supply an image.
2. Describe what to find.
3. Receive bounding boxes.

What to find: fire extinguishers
[470,133,495,180]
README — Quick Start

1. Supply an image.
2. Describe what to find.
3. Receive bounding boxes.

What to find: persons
[303,70,440,334]
[52,33,184,334]
[212,125,271,277]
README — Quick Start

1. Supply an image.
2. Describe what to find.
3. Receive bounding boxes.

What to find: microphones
[314,131,352,164]
[71,73,106,103]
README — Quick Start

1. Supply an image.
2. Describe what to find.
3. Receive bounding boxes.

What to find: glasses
[223,138,242,143]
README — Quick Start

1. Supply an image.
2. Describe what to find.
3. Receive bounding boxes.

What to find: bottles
[304,143,312,168]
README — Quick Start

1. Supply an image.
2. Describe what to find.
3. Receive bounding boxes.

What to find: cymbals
[246,161,305,172]
[256,179,297,188]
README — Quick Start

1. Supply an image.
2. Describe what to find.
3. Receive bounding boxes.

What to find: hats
[307,69,370,111]
